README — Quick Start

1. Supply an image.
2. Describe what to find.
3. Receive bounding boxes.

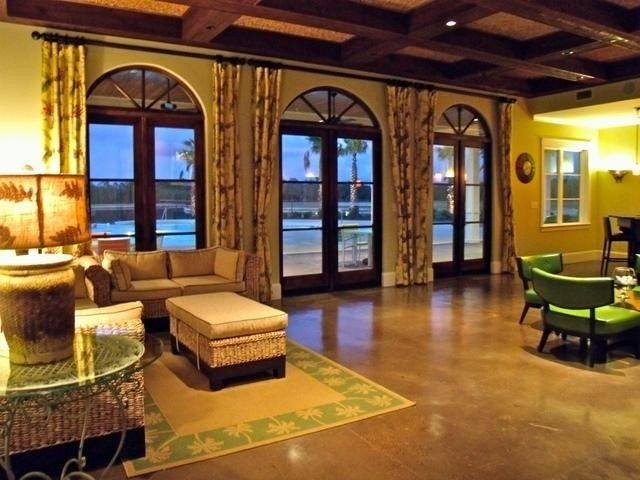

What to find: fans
[318,90,362,125]
[157,77,197,113]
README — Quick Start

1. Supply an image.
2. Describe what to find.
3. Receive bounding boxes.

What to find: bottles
[74,326,98,352]
[74,353,97,374]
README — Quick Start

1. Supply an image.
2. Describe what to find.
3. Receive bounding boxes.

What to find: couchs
[1,253,146,479]
[77,246,265,335]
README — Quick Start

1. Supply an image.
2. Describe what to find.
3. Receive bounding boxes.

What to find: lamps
[96,238,130,259]
[608,159,632,182]
[1,166,92,367]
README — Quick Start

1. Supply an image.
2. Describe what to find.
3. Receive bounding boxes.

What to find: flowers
[613,274,638,289]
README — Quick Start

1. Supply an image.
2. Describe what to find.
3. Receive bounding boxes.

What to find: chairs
[338,221,358,265]
[528,264,639,369]
[599,214,632,279]
[515,251,570,342]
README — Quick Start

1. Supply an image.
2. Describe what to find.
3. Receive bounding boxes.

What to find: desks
[345,231,371,268]
[1,334,164,480]
[612,288,640,312]
[609,215,640,267]
[164,292,292,392]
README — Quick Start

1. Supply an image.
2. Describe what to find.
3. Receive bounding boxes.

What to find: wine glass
[613,268,637,310]
[612,266,633,299]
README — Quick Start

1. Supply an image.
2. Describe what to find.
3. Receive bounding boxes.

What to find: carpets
[115,318,419,480]
